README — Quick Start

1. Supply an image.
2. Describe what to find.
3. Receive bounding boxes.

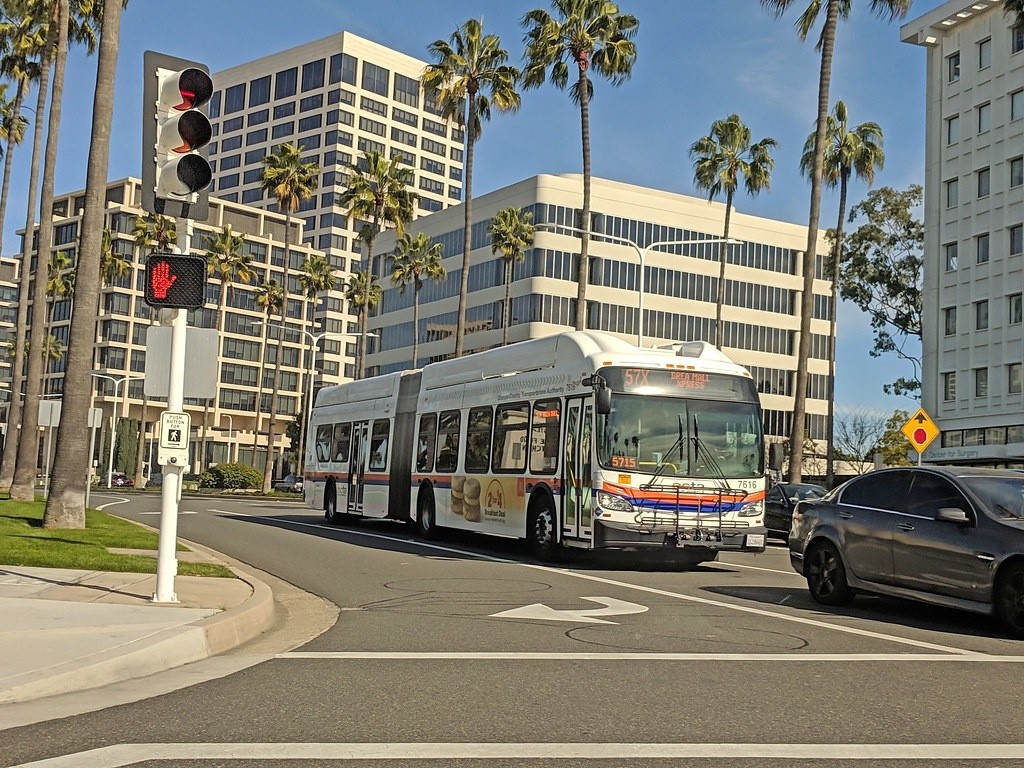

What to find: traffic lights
[143,250,210,314]
[152,65,214,206]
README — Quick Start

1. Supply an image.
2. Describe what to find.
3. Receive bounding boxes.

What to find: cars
[97,472,135,488]
[764,482,829,545]
[145,473,162,488]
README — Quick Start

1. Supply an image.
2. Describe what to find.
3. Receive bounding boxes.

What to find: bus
[299,330,784,566]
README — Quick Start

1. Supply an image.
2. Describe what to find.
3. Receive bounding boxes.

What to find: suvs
[788,464,1024,642]
[274,473,303,494]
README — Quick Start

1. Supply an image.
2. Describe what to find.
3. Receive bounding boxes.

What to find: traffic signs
[158,410,191,450]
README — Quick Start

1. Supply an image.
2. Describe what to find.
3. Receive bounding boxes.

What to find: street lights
[535,220,745,349]
[221,414,232,464]
[90,373,145,489]
[250,319,381,431]
